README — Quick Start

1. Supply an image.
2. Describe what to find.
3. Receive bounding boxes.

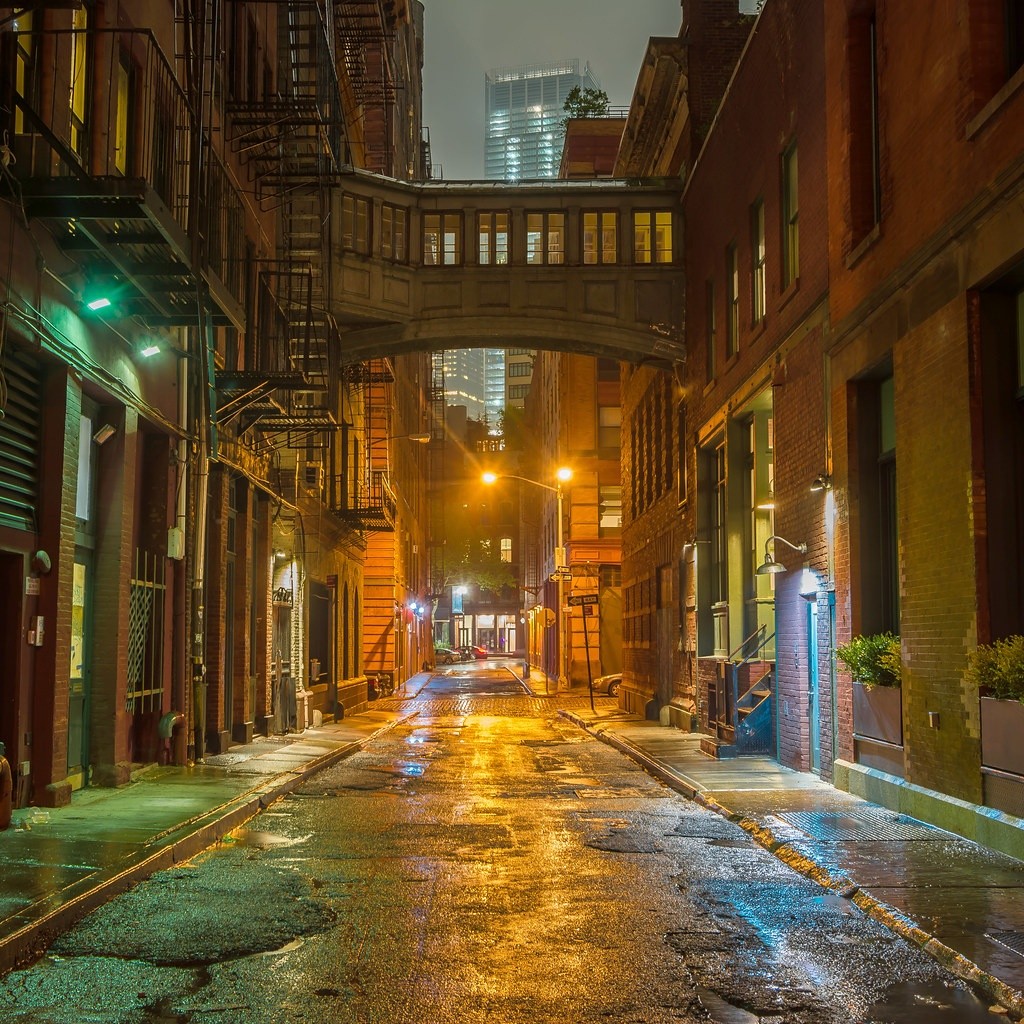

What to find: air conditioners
[306,467,325,490]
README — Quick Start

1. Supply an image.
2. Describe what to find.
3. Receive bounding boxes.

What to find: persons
[500,635,505,653]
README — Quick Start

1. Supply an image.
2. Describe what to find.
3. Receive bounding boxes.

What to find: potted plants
[828,630,902,746]
[960,635,1024,776]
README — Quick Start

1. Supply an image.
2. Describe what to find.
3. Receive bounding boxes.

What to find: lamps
[810,473,832,491]
[753,479,776,512]
[754,536,808,575]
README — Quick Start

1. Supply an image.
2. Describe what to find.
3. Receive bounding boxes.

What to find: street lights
[481,470,566,692]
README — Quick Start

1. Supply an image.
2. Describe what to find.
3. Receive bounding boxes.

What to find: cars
[456,646,488,660]
[434,648,464,665]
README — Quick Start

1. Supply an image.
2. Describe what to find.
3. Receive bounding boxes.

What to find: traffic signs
[549,573,572,582]
[566,593,599,608]
[557,566,569,573]
